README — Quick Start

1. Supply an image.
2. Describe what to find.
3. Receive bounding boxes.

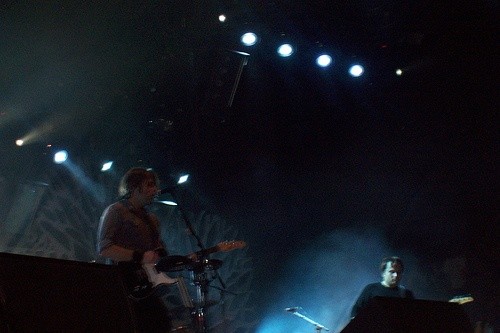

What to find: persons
[97,167,198,333]
[351,256,416,321]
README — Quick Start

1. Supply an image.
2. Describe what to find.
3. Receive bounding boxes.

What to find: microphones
[285,307,300,313]
[156,185,180,195]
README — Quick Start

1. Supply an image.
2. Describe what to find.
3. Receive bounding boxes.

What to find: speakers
[339,296,475,333]
[0,252,171,333]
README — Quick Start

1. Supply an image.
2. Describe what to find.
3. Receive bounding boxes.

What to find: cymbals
[167,300,220,317]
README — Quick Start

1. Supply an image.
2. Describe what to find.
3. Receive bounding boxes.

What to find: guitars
[117,239,248,304]
[448,294,474,304]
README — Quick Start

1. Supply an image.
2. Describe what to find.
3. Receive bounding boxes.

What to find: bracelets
[132,249,144,264]
